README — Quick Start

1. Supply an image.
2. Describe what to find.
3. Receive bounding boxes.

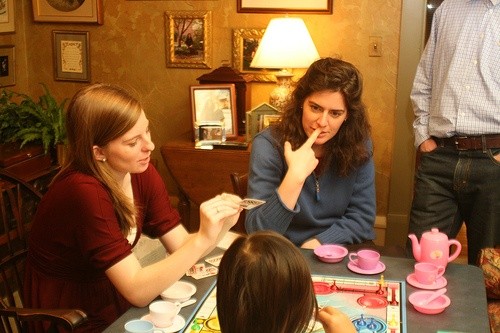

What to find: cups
[149,299,182,328]
[414,262,445,286]
[124,320,154,333]
[349,250,380,271]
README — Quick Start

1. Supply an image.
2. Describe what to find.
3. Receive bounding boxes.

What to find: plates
[406,273,447,289]
[347,259,386,275]
[140,315,185,333]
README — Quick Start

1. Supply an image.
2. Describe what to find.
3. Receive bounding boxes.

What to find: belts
[433,134,500,151]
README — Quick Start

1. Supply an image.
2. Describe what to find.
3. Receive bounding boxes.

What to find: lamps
[249,17,320,111]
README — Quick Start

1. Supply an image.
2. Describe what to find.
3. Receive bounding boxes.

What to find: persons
[404,0,500,268]
[23,82,243,333]
[245,57,380,248]
[217,230,357,333]
[179,32,193,48]
[201,128,221,139]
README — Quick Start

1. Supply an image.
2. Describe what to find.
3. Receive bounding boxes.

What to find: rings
[216,207,219,212]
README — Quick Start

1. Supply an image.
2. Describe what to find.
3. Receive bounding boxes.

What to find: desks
[102,248,491,333]
[159,128,252,235]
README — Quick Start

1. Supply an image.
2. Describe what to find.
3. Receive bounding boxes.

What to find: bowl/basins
[159,280,197,304]
[408,291,451,314]
[313,244,349,263]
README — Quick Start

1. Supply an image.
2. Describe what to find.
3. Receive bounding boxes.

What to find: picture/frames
[32,0,103,25]
[164,9,213,69]
[190,83,238,142]
[231,28,292,82]
[0,0,16,35]
[0,45,16,88]
[52,30,91,83]
[237,0,333,15]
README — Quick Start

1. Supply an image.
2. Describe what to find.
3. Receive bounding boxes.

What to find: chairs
[0,172,88,333]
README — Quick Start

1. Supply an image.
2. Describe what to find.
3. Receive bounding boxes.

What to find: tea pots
[407,227,462,274]
